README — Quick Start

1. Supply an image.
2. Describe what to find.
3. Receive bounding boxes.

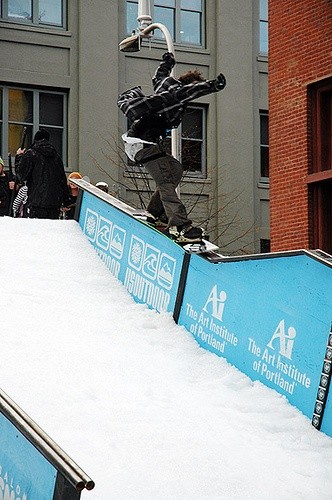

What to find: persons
[118,53,228,243]
[14,128,70,220]
[0,158,110,218]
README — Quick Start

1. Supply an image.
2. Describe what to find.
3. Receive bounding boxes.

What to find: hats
[96,182,109,187]
[68,172,82,179]
[0,158,5,167]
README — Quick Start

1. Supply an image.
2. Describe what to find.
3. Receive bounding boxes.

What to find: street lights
[118,0,182,200]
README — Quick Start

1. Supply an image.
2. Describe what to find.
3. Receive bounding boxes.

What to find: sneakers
[170,222,203,242]
[142,211,169,227]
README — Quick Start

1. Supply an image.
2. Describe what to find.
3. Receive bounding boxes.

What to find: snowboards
[131,207,221,254]
